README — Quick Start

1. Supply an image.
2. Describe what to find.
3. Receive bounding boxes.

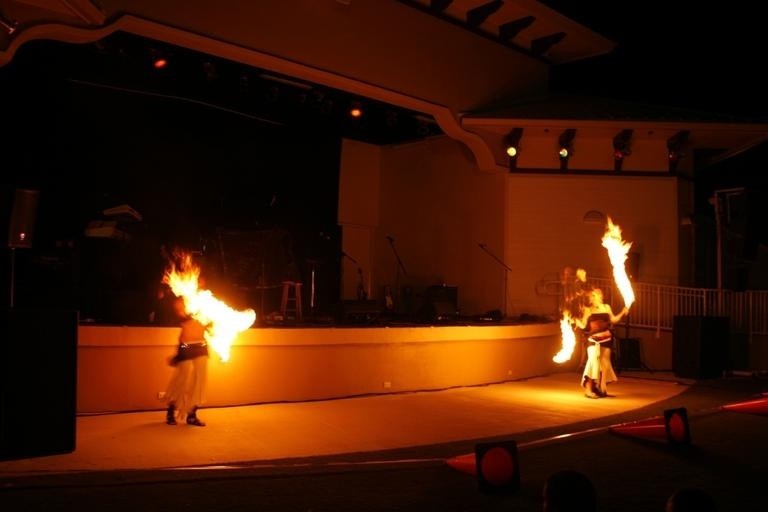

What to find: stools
[279,280,303,320]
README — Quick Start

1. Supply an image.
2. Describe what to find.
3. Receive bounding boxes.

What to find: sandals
[167,409,177,425]
[187,413,206,426]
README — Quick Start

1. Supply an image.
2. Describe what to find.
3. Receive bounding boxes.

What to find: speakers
[334,304,385,324]
[417,303,457,323]
[672,316,728,379]
[0,308,76,461]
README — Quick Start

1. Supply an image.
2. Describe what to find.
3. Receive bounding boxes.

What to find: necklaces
[594,305,600,309]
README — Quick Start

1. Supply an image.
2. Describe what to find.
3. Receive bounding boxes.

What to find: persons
[162,291,212,428]
[559,287,629,398]
[538,469,597,512]
[661,488,721,512]
